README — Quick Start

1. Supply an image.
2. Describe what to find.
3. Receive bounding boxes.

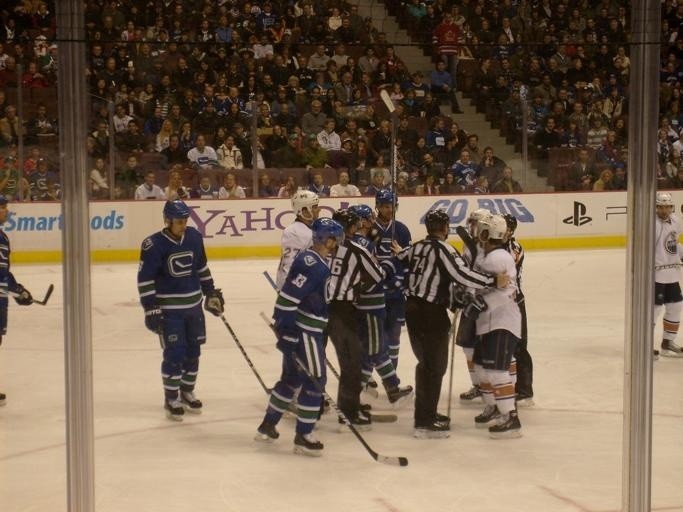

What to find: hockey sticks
[455,226,477,314]
[220,309,274,394]
[382,89,396,243]
[260,312,407,465]
[0,285,55,305]
[441,309,456,430]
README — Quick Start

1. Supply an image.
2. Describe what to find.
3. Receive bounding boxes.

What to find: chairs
[0,0,682,200]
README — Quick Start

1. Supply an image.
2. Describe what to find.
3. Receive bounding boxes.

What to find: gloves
[145,304,166,335]
[277,332,299,359]
[450,284,486,320]
[381,257,401,274]
[204,289,224,316]
[13,284,32,305]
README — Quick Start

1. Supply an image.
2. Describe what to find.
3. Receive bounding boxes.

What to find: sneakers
[415,413,450,430]
[258,420,282,439]
[165,397,184,415]
[295,432,323,450]
[654,339,683,354]
[179,391,202,408]
[339,408,370,424]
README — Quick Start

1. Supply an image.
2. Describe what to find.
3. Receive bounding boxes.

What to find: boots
[360,372,377,387]
[489,410,520,431]
[382,379,412,402]
[460,385,481,399]
[475,405,500,422]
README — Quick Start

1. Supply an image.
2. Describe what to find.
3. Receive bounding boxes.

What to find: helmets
[291,190,319,215]
[470,208,517,239]
[424,210,449,223]
[313,217,345,245]
[164,198,190,219]
[0,194,8,205]
[333,205,373,232]
[376,188,399,208]
[656,192,674,206]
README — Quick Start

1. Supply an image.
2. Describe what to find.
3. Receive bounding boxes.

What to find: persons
[349,205,413,403]
[0,195,32,401]
[136,200,224,416]
[323,208,387,424]
[653,193,683,361]
[369,188,412,371]
[389,209,533,430]
[257,217,346,451]
[275,188,322,292]
